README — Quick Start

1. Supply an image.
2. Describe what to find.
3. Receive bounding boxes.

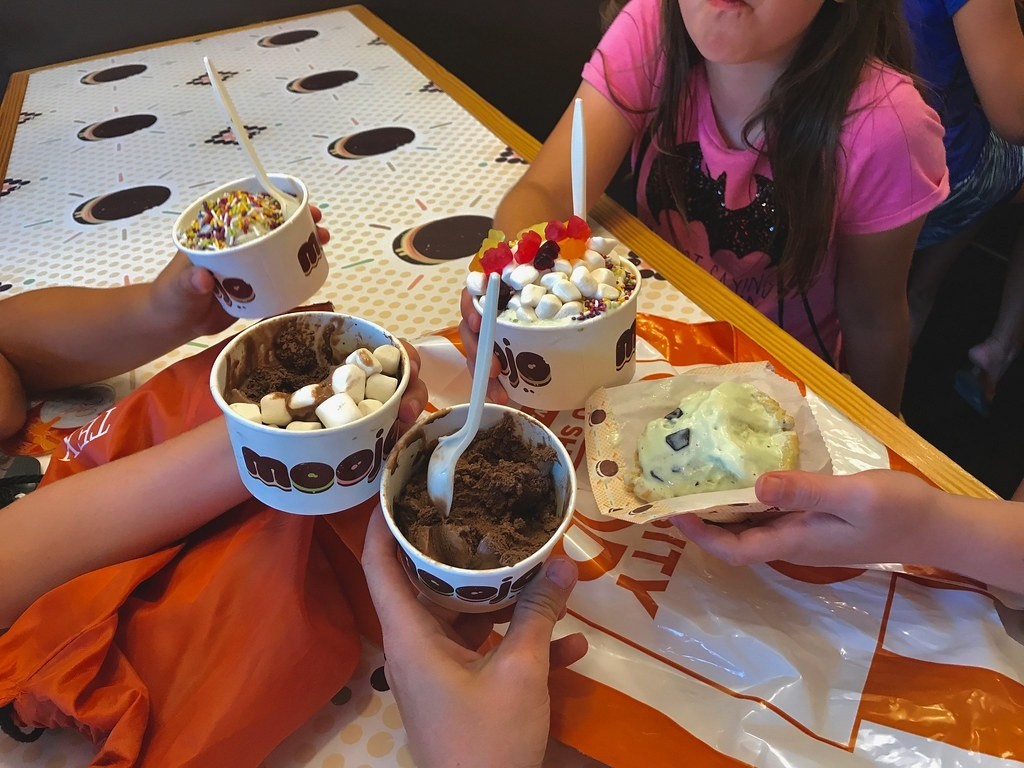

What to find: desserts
[630,380,800,504]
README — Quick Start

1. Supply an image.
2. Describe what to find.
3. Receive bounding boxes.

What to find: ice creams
[227,333,401,429]
[395,415,563,567]
[176,190,284,250]
[470,216,639,325]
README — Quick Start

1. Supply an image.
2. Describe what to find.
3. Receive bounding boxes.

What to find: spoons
[425,272,500,514]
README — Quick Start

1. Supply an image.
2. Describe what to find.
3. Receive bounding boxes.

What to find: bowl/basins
[378,401,579,616]
[473,249,641,411]
[171,173,330,320]
[209,311,410,514]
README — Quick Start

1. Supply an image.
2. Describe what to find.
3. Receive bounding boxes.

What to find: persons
[494,1,949,420]
[668,469,1023,598]
[361,505,589,768]
[0,206,428,641]
[899,1,1023,247]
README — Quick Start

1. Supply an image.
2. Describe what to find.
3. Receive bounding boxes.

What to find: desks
[0,0,1024,767]
